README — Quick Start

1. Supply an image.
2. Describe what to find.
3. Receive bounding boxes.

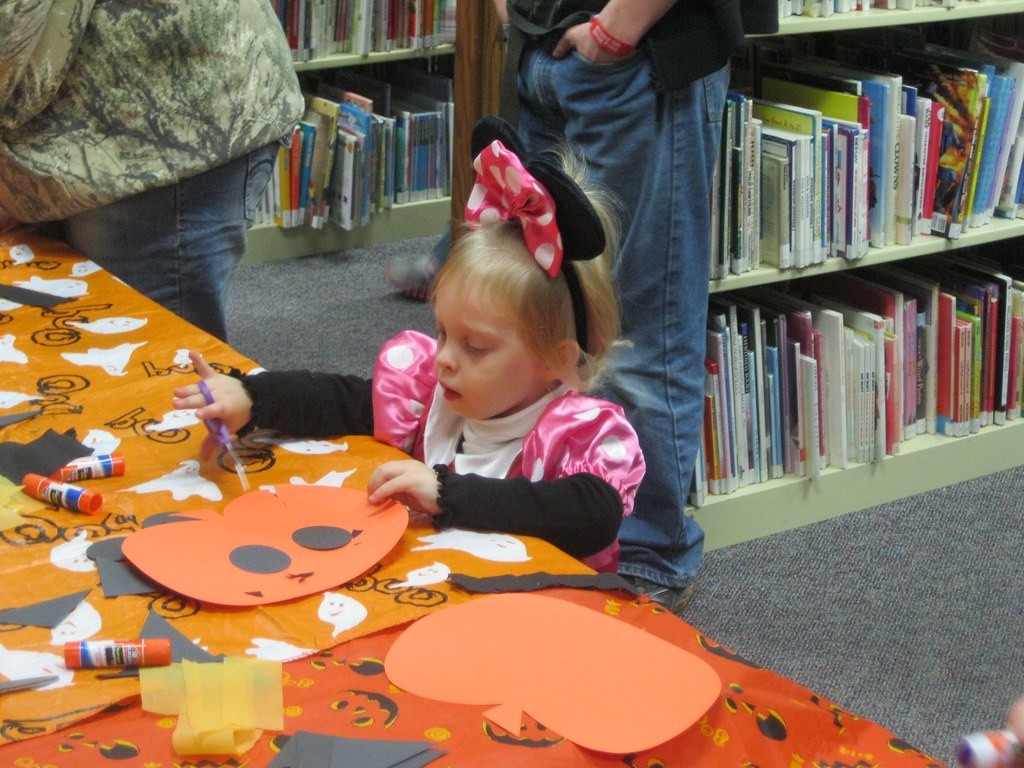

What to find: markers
[955,730,1023,768]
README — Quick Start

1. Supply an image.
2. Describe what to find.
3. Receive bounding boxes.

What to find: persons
[172,116,645,575]
[393,229,454,302]
[476,0,748,615]
[0,0,307,345]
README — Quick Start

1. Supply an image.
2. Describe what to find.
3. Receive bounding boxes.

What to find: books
[244,2,457,229]
[688,1,1024,511]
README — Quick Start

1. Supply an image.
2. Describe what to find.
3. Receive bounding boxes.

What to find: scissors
[0,411,41,428]
[196,380,250,492]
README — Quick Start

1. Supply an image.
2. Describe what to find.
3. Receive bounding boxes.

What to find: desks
[1,231,942,768]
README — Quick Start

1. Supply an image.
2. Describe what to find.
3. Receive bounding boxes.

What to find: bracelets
[590,16,635,55]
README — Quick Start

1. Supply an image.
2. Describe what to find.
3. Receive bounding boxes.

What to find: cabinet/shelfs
[453,0,1024,553]
[242,43,453,264]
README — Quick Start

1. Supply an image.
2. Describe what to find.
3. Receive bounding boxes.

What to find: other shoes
[619,573,696,614]
[384,258,439,300]
